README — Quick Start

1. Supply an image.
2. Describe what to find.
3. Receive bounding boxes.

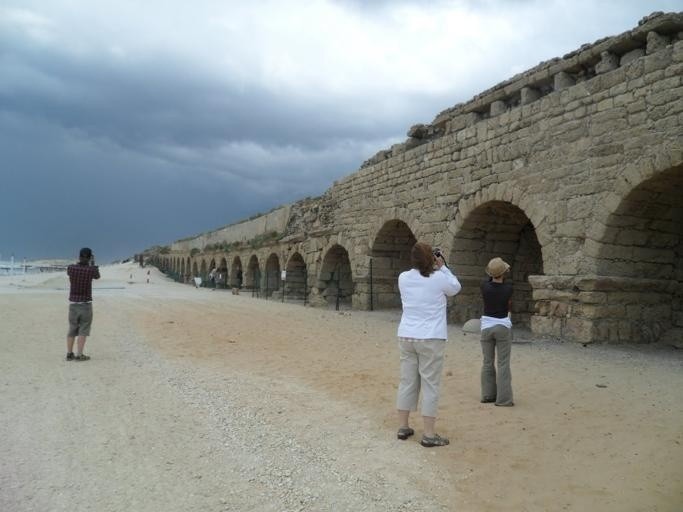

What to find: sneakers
[67,352,75,361]
[481,396,514,406]
[422,433,449,447]
[75,354,90,360]
[397,427,414,439]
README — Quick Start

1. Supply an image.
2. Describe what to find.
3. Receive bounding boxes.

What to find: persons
[394,240,461,447]
[479,257,516,407]
[66,246,99,359]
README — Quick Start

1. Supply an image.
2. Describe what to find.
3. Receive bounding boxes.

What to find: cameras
[432,248,441,257]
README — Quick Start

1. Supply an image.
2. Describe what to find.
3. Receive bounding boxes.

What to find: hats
[80,248,91,257]
[486,257,509,277]
